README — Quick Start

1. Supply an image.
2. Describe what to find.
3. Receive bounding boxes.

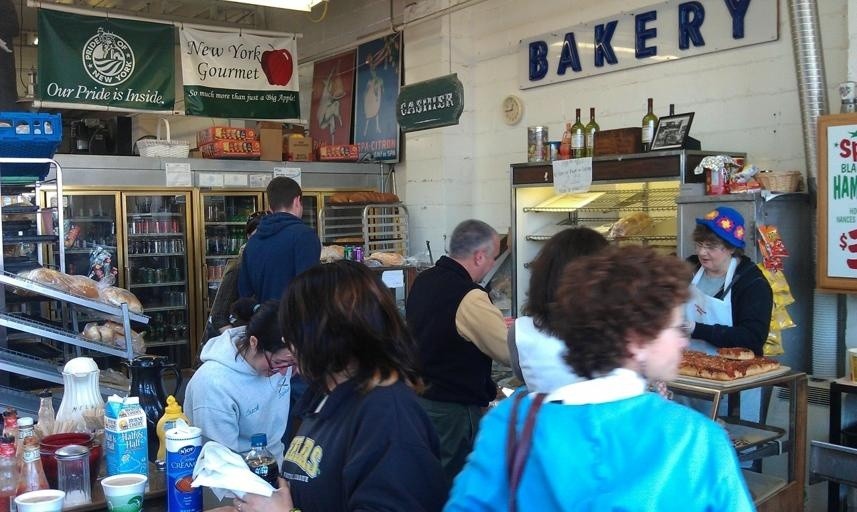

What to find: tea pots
[52,356,106,433]
[118,354,181,464]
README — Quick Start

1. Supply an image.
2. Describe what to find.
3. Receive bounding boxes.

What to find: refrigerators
[194,171,323,372]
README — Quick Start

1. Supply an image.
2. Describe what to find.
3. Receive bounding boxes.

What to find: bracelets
[287,508,302,512]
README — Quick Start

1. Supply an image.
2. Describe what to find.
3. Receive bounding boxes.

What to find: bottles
[0,444,18,509]
[207,262,226,280]
[18,416,32,432]
[15,437,51,497]
[57,444,90,507]
[174,266,183,281]
[203,194,254,223]
[139,267,171,283]
[160,288,187,306]
[207,226,249,255]
[356,247,361,257]
[0,408,17,440]
[560,123,573,161]
[641,99,659,153]
[146,313,189,341]
[243,433,278,491]
[528,126,548,163]
[569,109,586,159]
[346,246,354,260]
[586,108,600,157]
[670,104,674,116]
[126,199,187,254]
[36,391,56,439]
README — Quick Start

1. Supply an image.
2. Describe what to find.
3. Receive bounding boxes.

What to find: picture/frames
[354,30,403,166]
[651,112,695,151]
[818,113,857,293]
[310,46,355,156]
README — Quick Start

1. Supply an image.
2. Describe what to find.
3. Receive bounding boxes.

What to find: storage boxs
[0,112,63,177]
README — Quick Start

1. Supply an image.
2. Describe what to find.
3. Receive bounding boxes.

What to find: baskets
[754,172,802,193]
[136,118,190,158]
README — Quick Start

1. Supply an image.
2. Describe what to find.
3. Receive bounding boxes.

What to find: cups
[14,488,65,512]
[100,472,148,512]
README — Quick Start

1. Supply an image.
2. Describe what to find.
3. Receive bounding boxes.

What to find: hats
[697,207,746,247]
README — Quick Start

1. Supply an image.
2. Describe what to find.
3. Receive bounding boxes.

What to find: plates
[0,269,151,324]
[0,311,148,362]
[670,364,792,388]
[714,416,786,453]
[0,385,62,422]
[740,468,788,507]
[0,347,130,399]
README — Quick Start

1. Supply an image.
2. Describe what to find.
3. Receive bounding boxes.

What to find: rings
[236,502,243,511]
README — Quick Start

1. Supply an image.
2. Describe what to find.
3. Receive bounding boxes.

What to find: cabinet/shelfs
[195,189,264,342]
[301,191,374,256]
[511,152,745,317]
[679,195,815,373]
[2,158,67,395]
[1,277,147,417]
[52,154,394,189]
[668,372,809,512]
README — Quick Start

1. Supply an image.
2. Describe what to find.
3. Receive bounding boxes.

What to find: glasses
[262,349,296,373]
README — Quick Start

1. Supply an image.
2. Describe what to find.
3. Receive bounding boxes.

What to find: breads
[84,323,146,356]
[679,346,780,382]
[608,214,655,244]
[35,267,70,293]
[69,274,103,302]
[105,287,143,316]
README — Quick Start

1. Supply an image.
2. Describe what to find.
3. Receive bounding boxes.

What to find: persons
[507,227,614,390]
[441,244,757,512]
[679,207,774,361]
[195,211,276,339]
[236,176,323,309]
[200,261,450,512]
[405,219,512,476]
[182,297,303,504]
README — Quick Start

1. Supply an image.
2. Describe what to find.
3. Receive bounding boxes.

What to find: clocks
[501,96,522,127]
